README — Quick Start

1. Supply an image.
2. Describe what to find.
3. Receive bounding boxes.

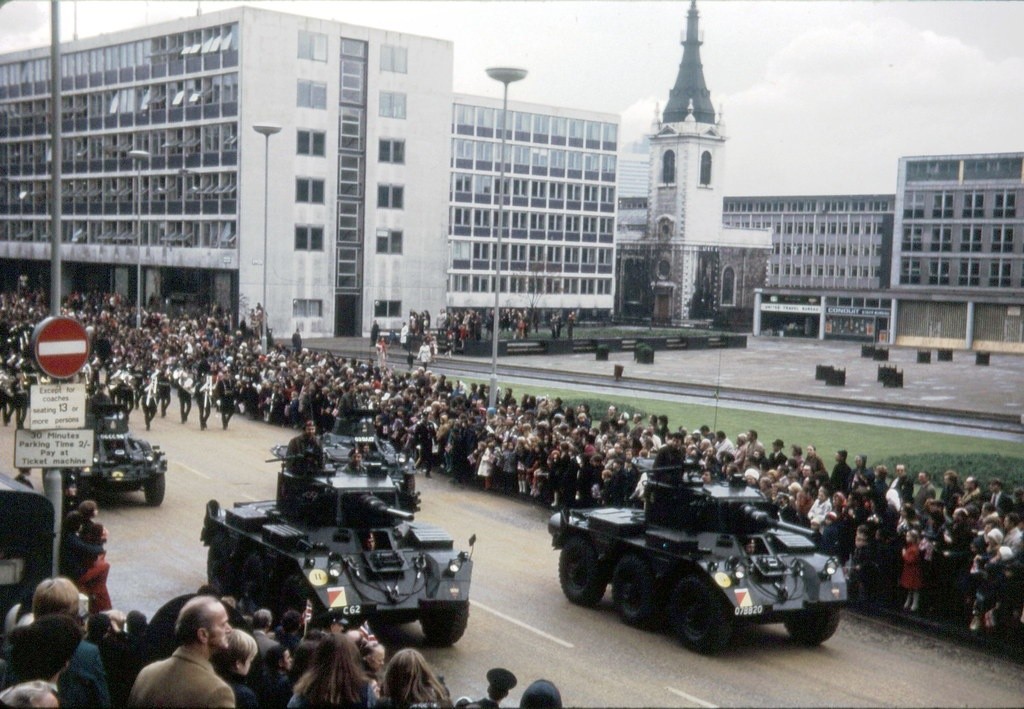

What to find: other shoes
[551,501,557,506]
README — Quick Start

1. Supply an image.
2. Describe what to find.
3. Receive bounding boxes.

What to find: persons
[0,279,1024,709]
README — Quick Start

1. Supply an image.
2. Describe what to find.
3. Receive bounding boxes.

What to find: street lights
[487,68,528,408]
[128,150,152,328]
[253,125,283,356]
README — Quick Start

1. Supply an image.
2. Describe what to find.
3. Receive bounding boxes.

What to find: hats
[301,419,315,429]
[422,411,429,416]
[988,528,1003,544]
[745,469,759,480]
[772,439,784,448]
[486,667,517,691]
[691,429,700,436]
[361,442,369,446]
[909,528,919,537]
[347,448,363,457]
[738,433,746,441]
[999,546,1013,560]
[63,468,76,489]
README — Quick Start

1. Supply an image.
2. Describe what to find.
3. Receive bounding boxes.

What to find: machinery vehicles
[200,396,477,647]
[546,457,849,656]
[63,404,166,505]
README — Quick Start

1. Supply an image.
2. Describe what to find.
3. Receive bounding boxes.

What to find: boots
[904,592,919,611]
[264,411,269,422]
[519,481,526,493]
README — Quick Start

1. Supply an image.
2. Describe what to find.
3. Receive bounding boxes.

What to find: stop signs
[34,317,90,380]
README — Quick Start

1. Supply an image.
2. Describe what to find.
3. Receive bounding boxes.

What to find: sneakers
[970,616,980,630]
